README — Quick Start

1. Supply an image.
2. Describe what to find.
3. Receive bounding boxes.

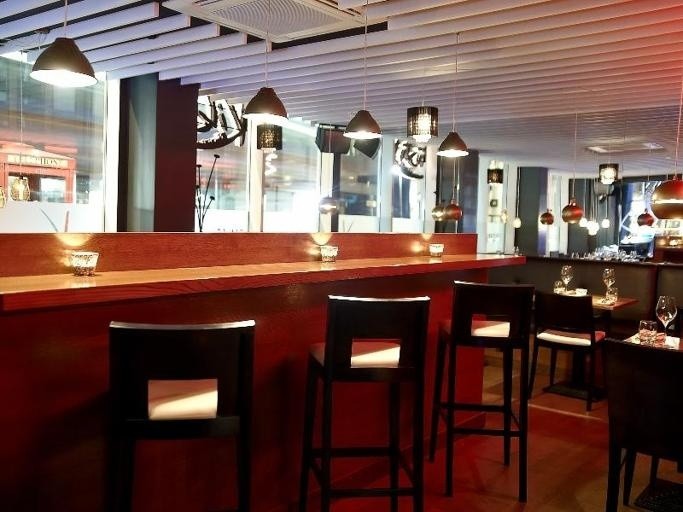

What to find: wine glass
[655,295,677,347]
[602,268,615,303]
[561,266,572,293]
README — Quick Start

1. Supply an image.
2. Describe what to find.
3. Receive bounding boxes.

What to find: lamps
[241,23,289,124]
[434,147,468,225]
[403,19,441,142]
[435,30,469,158]
[318,154,339,216]
[562,118,584,224]
[342,0,383,139]
[7,50,32,202]
[514,163,522,227]
[27,2,99,90]
[253,119,288,155]
[540,167,555,226]
[578,144,617,236]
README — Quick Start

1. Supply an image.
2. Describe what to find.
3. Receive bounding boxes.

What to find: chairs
[426,275,538,508]
[299,288,434,512]
[516,285,682,512]
[99,308,258,510]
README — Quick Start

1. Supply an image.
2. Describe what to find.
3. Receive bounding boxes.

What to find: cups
[638,320,657,343]
[70,251,99,277]
[429,244,444,258]
[553,281,563,294]
[320,245,337,263]
[575,288,587,296]
[571,246,637,262]
[606,287,618,301]
